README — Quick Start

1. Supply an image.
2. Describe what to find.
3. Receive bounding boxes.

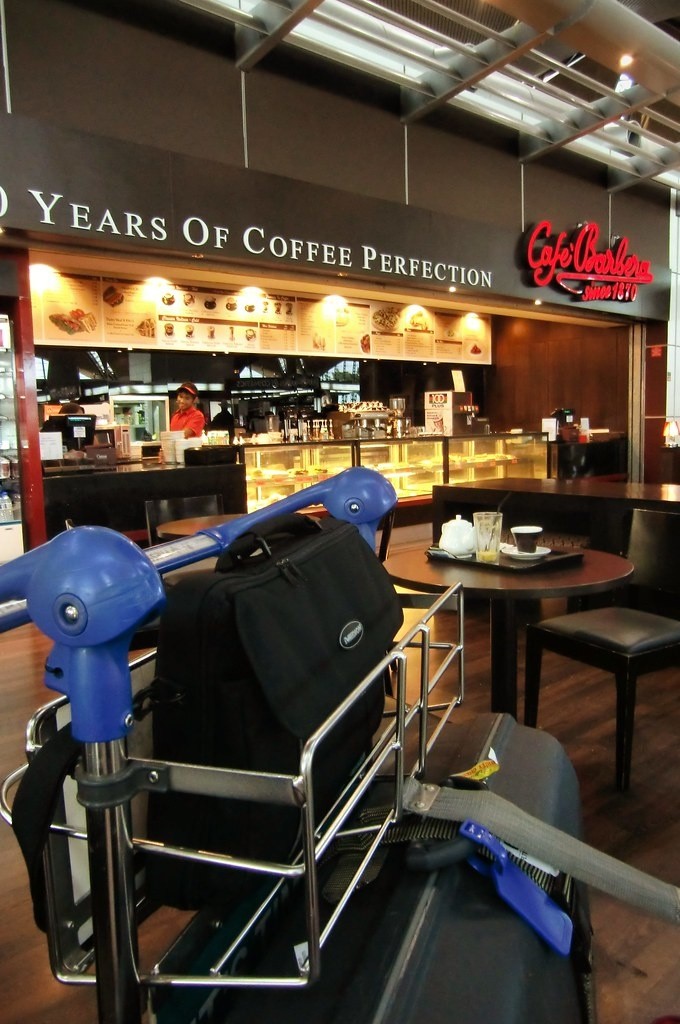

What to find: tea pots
[439,515,475,559]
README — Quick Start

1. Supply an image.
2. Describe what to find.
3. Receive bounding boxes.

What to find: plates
[371,311,400,331]
[501,546,551,561]
[174,440,201,464]
[161,431,184,465]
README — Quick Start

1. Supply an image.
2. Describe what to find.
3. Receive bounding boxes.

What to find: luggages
[147,713,589,1023]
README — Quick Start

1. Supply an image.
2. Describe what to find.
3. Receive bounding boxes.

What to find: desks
[382,548,634,724]
[157,512,322,542]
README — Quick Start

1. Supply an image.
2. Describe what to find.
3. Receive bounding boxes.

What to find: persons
[171,383,205,437]
[60,403,98,446]
[212,401,235,443]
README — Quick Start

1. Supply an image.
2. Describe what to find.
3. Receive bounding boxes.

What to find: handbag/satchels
[152,513,404,910]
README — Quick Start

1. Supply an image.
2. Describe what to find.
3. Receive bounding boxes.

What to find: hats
[176,383,198,395]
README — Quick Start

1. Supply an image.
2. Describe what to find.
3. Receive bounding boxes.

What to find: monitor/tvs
[550,408,575,427]
[49,414,96,450]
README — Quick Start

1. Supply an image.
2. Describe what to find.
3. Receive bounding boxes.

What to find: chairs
[523,508,680,790]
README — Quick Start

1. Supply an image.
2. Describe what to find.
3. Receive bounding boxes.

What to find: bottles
[2,492,14,522]
[0,495,6,523]
[123,429,130,456]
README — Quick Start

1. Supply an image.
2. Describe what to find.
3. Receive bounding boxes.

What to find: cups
[510,526,543,554]
[185,325,194,337]
[274,302,282,314]
[245,329,255,341]
[207,327,215,339]
[286,304,292,315]
[164,324,174,336]
[472,512,503,565]
